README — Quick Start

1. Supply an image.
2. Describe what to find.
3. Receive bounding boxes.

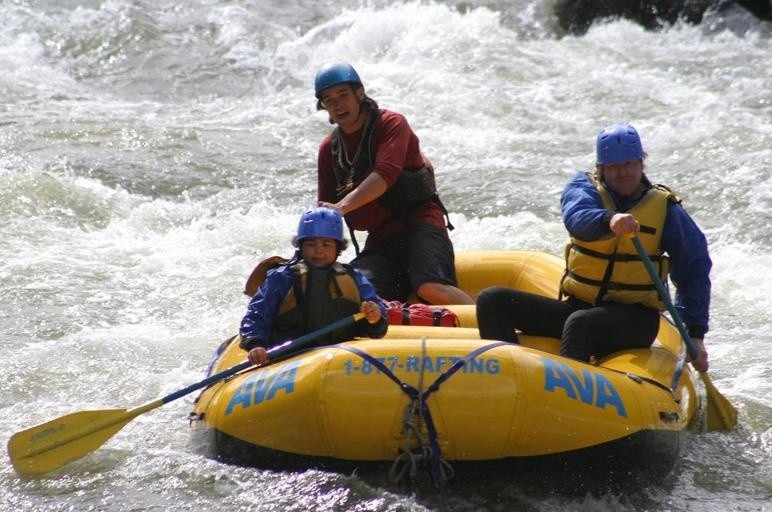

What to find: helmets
[314,59,364,101]
[596,123,643,164]
[296,207,345,242]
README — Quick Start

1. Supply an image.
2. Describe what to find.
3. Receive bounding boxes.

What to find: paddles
[8,311,366,475]
[625,220,738,433]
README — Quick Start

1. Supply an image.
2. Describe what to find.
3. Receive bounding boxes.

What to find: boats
[188,246,702,501]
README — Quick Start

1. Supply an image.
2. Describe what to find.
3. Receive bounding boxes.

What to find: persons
[477,125,713,364]
[291,61,475,305]
[396,121,413,136]
[238,207,388,366]
